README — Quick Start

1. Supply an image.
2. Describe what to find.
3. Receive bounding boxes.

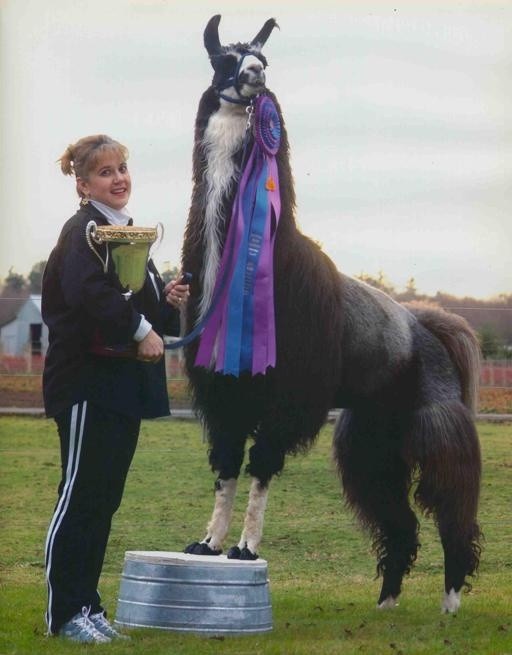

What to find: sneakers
[61,604,133,645]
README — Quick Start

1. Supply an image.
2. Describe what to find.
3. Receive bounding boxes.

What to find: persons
[40,133,192,646]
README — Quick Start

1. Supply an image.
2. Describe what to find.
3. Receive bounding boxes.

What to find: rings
[176,298,182,304]
